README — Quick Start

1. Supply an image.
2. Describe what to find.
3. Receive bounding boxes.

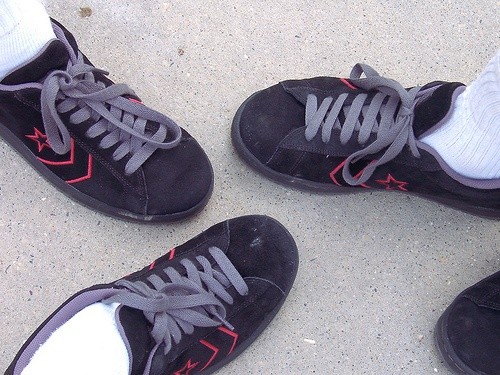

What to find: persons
[0,0,215,224]
[0,211,300,375]
[229,47,500,374]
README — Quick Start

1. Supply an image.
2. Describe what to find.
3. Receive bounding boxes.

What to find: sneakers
[230,63,499,223]
[5,212,298,374]
[1,16,215,224]
[433,269,499,375]
